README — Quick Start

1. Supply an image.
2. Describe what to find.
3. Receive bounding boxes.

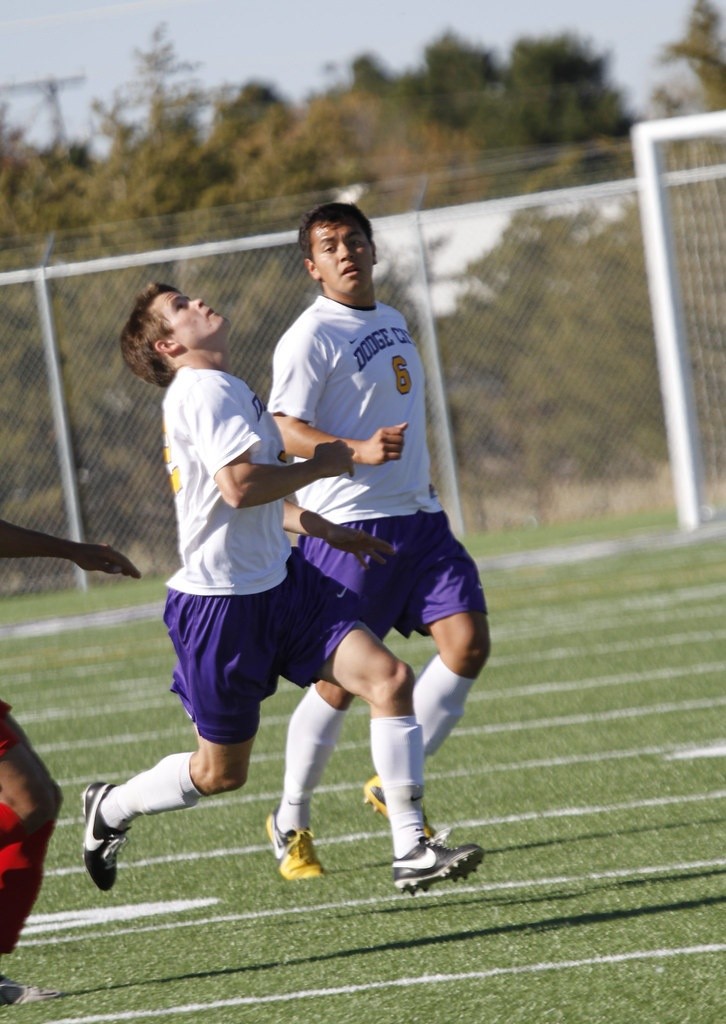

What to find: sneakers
[362,776,433,839]
[82,782,132,890]
[392,838,483,896]
[265,805,326,879]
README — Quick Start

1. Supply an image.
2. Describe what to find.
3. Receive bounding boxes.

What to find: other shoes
[0,973,62,1009]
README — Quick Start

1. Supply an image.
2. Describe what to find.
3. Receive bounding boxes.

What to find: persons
[0,522,142,1008]
[264,203,492,881]
[79,284,487,897]
